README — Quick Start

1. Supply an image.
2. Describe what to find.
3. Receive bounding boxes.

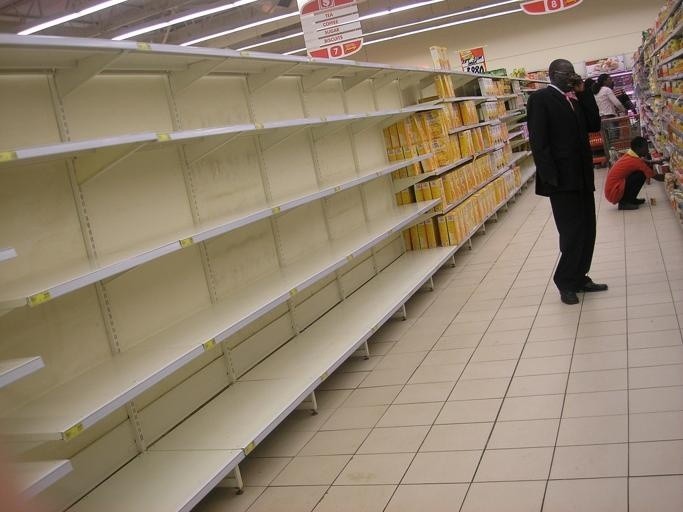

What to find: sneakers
[619,198,645,210]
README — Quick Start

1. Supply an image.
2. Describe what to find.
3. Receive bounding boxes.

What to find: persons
[584,73,637,169]
[604,135,668,211]
[526,59,608,305]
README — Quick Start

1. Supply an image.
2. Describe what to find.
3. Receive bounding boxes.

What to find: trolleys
[599,111,643,169]
[584,118,611,169]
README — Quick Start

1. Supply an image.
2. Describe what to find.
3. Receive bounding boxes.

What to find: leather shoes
[576,280,607,292]
[561,288,578,303]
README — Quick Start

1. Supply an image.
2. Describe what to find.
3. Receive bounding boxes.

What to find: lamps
[12,1,125,36]
[96,0,255,41]
[169,6,300,46]
[221,0,535,56]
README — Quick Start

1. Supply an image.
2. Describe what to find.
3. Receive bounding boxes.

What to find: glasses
[556,70,576,77]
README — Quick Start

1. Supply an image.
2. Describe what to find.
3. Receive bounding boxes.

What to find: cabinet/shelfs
[502,53,645,211]
[47,42,318,492]
[244,49,503,361]
[628,1,683,235]
[165,50,376,414]
[1,33,244,512]
